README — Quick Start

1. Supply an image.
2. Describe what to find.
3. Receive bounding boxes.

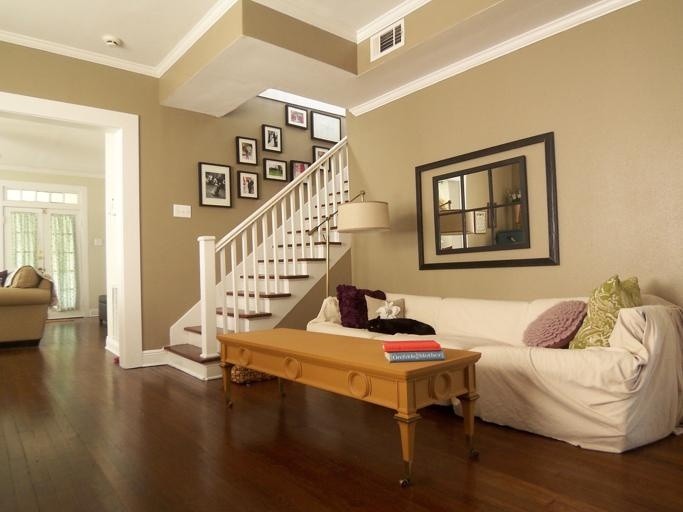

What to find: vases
[511,203,521,224]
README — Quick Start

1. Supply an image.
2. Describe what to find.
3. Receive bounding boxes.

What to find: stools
[96,294,108,324]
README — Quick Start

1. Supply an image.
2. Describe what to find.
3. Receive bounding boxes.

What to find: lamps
[303,189,392,297]
[435,198,487,237]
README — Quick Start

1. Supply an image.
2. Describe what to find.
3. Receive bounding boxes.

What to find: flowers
[503,187,521,204]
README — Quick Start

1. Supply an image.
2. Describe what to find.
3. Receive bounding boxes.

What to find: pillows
[334,283,387,330]
[522,298,587,351]
[362,293,407,322]
[564,272,644,354]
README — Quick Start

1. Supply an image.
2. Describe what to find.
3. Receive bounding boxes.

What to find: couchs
[303,286,682,456]
[0,260,59,350]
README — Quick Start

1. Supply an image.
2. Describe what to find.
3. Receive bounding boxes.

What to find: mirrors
[414,131,561,272]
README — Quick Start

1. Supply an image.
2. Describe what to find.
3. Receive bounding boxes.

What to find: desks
[215,326,485,490]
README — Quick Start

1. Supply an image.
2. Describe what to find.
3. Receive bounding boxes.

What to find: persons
[248,178,253,193]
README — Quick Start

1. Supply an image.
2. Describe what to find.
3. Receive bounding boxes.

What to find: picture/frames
[196,99,343,210]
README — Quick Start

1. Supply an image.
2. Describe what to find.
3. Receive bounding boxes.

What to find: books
[381,340,441,351]
[385,351,444,361]
